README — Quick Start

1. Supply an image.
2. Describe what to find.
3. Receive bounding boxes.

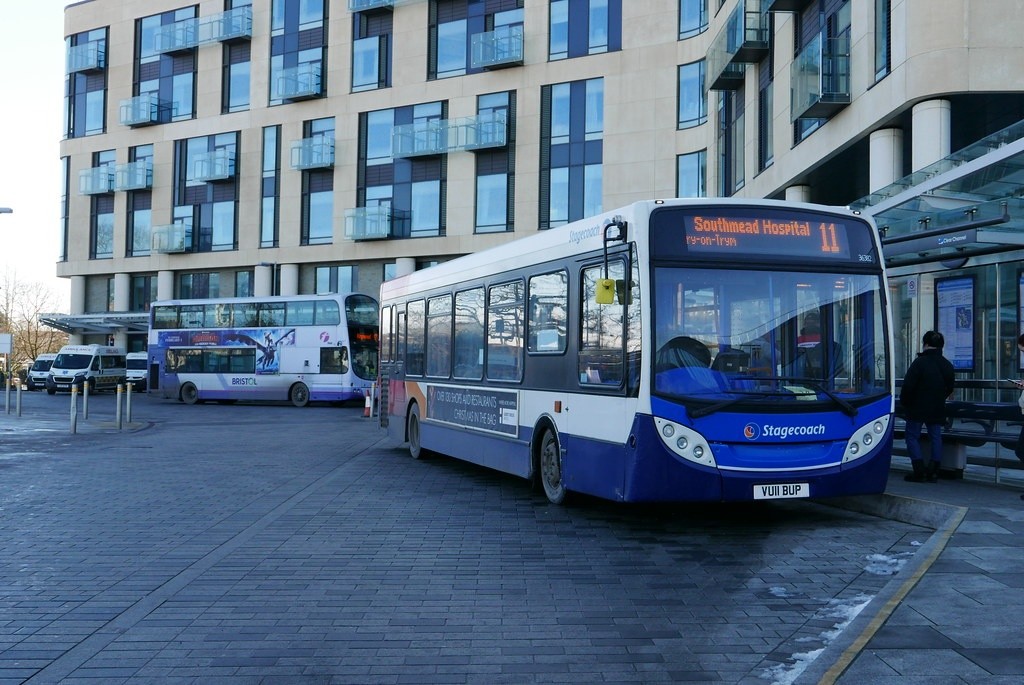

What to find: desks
[892,394,1024,470]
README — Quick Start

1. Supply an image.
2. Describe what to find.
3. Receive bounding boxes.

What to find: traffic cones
[360,389,377,418]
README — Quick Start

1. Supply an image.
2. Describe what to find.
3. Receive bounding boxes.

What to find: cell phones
[1007,379,1023,387]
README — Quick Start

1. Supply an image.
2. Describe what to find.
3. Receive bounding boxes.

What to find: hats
[804,312,820,321]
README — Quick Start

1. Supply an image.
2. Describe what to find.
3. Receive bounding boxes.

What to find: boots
[904,458,923,482]
[922,459,941,483]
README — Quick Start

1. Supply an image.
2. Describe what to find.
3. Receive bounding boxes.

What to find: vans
[26,352,64,391]
[125,351,148,392]
[45,343,127,396]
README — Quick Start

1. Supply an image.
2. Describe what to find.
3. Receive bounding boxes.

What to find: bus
[145,291,380,409]
[374,194,897,510]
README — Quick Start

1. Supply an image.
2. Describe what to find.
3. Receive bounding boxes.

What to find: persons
[1015,334,1024,500]
[897,331,955,483]
[742,323,781,376]
[797,314,849,382]
